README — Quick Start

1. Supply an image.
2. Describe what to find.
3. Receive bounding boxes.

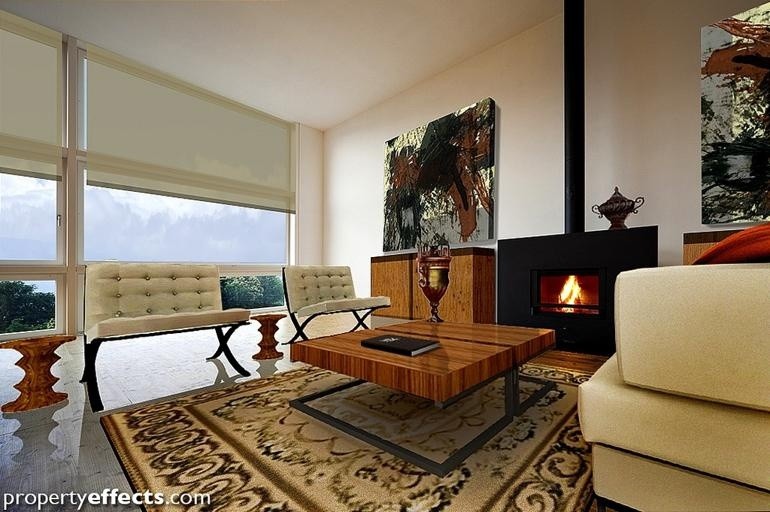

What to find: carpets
[96,363,635,512]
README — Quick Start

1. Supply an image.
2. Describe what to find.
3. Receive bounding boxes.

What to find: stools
[252,313,286,360]
[0,334,77,416]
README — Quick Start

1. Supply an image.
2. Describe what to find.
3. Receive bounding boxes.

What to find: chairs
[281,259,395,346]
[79,263,251,415]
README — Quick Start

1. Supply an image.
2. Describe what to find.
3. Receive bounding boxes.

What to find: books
[361,334,441,358]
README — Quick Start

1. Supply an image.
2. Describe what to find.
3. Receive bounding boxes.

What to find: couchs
[578,261,770,512]
